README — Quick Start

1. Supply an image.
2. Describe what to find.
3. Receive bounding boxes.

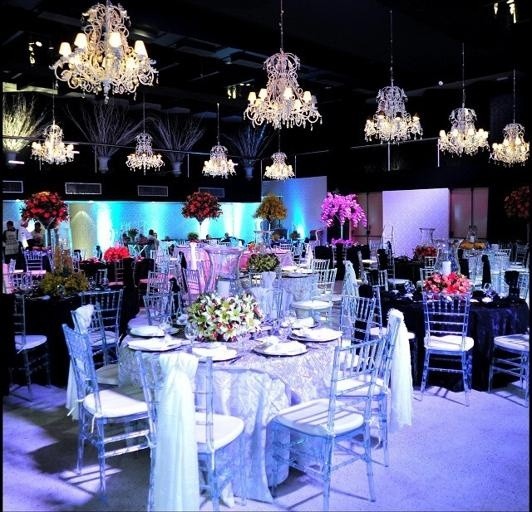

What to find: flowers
[319,188,366,229]
[179,191,223,224]
[18,192,69,228]
[253,193,288,221]
[505,187,531,221]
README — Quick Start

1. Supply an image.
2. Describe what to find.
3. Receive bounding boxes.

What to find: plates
[128,317,342,363]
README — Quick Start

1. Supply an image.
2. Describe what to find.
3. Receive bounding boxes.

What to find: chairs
[68,302,140,456]
[62,325,163,506]
[134,351,244,510]
[271,334,383,510]
[327,313,399,466]
[420,291,477,408]
[3,234,530,402]
[487,333,529,407]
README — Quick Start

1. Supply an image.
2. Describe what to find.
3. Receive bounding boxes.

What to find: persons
[96,246,102,261]
[169,245,188,329]
[2,219,51,295]
[269,230,286,249]
[148,230,156,242]
[221,233,230,242]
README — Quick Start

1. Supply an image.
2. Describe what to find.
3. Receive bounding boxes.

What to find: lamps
[34,94,80,170]
[124,86,165,177]
[243,1,325,132]
[265,129,297,180]
[487,67,531,168]
[363,3,423,143]
[50,4,161,106]
[439,41,491,158]
[202,102,238,180]
